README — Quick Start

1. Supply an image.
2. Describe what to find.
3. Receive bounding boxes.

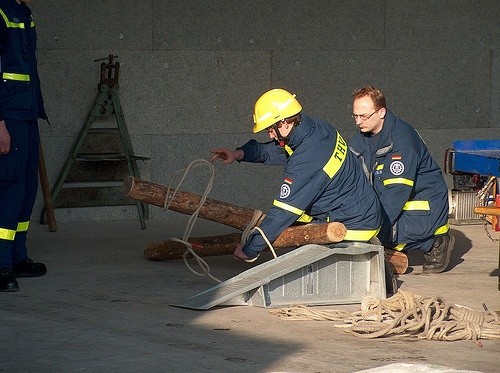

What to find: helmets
[253,89,302,134]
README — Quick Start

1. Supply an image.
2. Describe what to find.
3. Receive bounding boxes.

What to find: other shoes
[369,237,397,295]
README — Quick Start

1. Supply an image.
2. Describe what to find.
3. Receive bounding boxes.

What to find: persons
[0,1,50,293]
[345,85,456,273]
[209,88,387,263]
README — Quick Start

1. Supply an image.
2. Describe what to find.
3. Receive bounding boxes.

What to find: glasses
[351,106,383,121]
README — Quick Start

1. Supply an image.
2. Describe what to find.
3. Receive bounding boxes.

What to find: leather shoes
[422,233,456,274]
[15,262,47,277]
[0,272,20,292]
